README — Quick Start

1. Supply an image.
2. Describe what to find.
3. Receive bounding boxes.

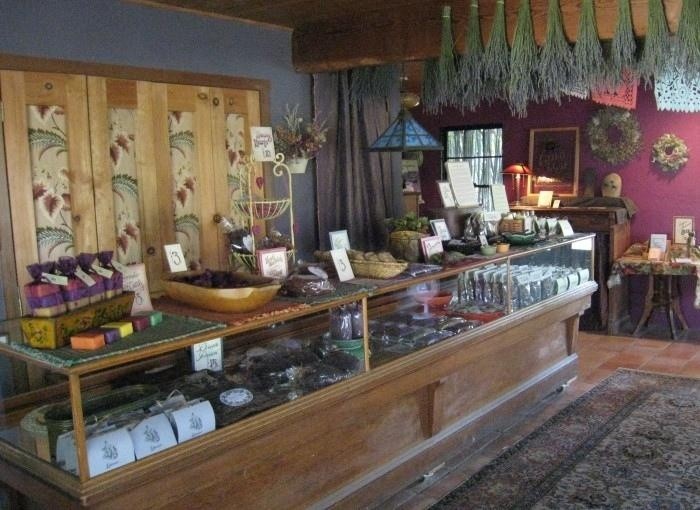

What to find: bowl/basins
[158,269,282,314]
[316,258,408,280]
[324,332,363,349]
[417,292,454,307]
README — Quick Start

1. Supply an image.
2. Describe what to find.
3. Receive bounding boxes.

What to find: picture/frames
[527,127,581,198]
[672,215,695,247]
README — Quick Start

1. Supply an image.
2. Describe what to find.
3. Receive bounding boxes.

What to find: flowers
[271,103,329,161]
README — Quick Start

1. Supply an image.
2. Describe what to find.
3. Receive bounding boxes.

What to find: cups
[495,243,510,253]
[480,245,496,256]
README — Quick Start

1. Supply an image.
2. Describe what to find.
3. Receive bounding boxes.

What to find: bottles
[516,210,539,234]
[686,231,696,257]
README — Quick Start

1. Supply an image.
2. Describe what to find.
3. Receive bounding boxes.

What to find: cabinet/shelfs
[0,230,601,510]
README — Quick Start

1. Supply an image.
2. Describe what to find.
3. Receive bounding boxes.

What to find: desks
[607,244,699,340]
[510,205,634,335]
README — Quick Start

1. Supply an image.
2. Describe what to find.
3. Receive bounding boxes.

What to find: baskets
[233,250,293,272]
[349,259,407,279]
[232,199,289,220]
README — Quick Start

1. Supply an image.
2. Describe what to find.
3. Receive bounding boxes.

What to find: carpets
[423,366,699,508]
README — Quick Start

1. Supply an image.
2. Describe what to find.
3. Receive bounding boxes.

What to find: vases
[283,156,308,175]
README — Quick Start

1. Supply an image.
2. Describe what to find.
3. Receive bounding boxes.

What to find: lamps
[498,160,533,207]
[363,32,444,154]
